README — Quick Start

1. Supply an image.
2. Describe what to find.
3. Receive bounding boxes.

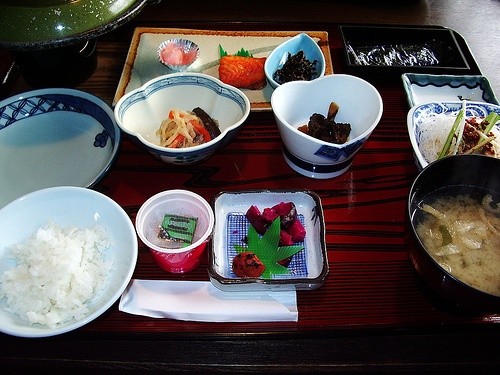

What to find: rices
[0,218,112,328]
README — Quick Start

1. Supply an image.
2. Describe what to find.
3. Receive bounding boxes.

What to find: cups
[135,189,215,273]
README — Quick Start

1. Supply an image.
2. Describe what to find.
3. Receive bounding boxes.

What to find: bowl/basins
[0,186,139,338]
[0,88,120,209]
[407,100,500,170]
[116,72,250,162]
[207,188,331,292]
[271,73,384,179]
[264,32,326,88]
[405,154,500,313]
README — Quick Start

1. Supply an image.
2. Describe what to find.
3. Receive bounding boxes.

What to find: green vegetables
[235,217,305,279]
[219,45,253,57]
[437,111,500,158]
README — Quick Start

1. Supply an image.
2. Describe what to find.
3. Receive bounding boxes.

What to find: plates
[0,0,146,49]
[401,73,500,110]
[113,24,334,112]
